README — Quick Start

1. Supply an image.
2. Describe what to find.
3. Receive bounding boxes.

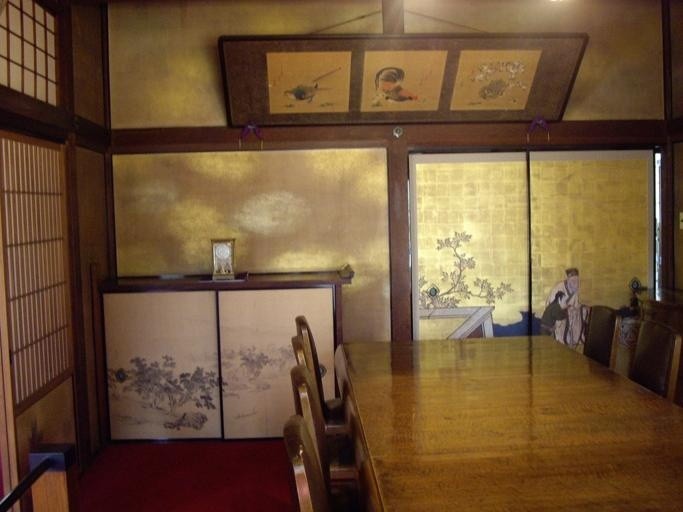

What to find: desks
[333,335,682,510]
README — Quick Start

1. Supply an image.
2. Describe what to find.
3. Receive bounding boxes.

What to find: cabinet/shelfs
[96,263,355,445]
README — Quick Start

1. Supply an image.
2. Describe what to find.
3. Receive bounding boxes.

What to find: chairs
[581,297,681,409]
[283,312,369,512]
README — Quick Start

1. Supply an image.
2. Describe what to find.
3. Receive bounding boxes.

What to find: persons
[539,290,570,339]
[542,267,590,351]
